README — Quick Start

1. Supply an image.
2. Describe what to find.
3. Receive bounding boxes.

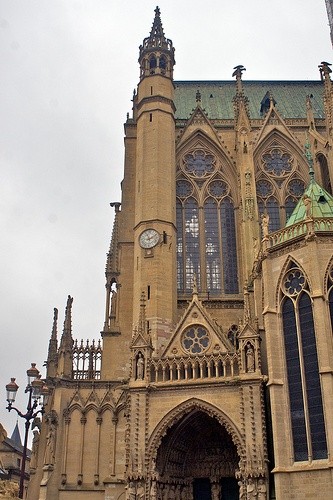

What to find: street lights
[4,363,45,499]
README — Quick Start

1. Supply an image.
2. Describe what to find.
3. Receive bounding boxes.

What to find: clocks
[138,229,161,249]
